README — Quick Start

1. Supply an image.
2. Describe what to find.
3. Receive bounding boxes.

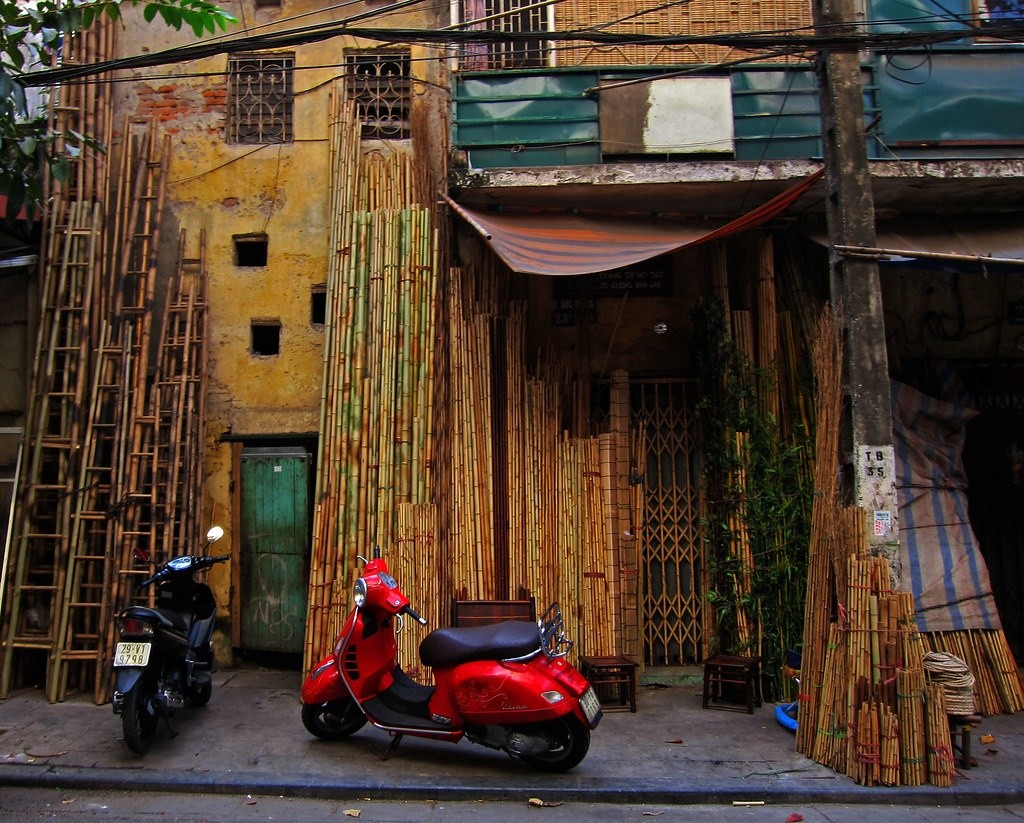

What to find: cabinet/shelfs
[450,596,538,625]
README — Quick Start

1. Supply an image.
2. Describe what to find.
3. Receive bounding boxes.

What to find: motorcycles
[111,526,231,752]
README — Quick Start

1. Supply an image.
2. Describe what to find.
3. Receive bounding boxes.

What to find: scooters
[298,545,605,771]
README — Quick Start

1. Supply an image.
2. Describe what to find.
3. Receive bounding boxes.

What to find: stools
[702,655,762,715]
[579,654,640,714]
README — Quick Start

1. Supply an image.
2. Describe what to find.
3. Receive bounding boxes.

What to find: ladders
[0,199,210,705]
